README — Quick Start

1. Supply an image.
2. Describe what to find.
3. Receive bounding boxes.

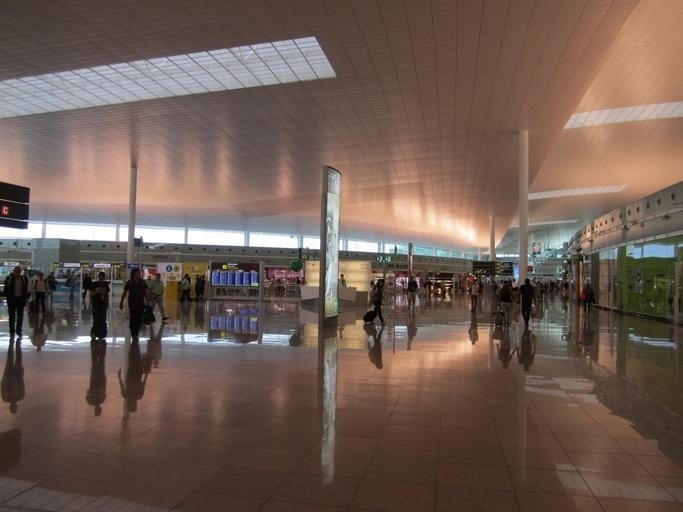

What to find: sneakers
[17,331,22,336]
[91,327,96,337]
[133,335,138,341]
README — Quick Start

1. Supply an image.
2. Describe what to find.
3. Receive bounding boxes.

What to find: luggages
[363,310,377,322]
[28,301,35,314]
[493,304,505,326]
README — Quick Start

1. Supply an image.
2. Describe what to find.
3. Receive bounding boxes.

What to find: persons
[515,278,536,331]
[406,312,416,349]
[147,321,167,369]
[84,339,106,416]
[338,274,346,287]
[0,265,208,344]
[400,272,574,314]
[583,319,593,347]
[0,338,25,412]
[367,324,385,369]
[498,282,514,327]
[468,313,478,344]
[116,339,149,412]
[368,278,386,324]
[581,282,594,313]
[515,330,536,371]
[495,327,517,369]
[29,312,47,351]
[213,273,304,297]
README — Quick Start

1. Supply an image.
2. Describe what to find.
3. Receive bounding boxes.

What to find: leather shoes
[162,317,169,320]
